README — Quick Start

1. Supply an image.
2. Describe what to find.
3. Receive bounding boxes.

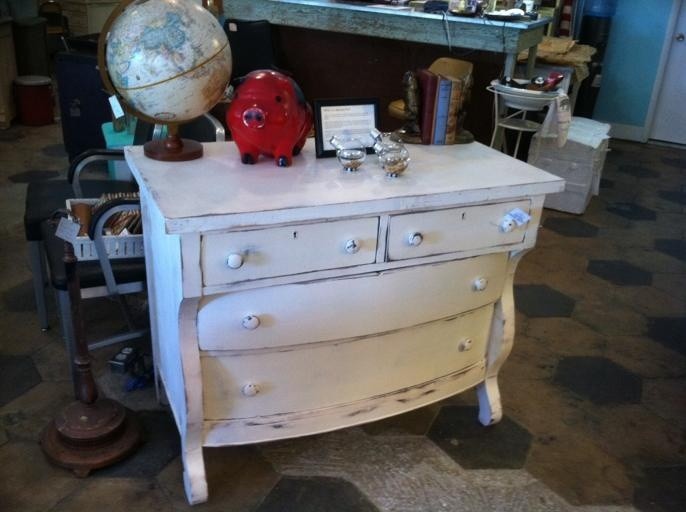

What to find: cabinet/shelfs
[124,137,567,507]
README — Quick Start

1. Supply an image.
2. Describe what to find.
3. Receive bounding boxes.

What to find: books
[88,191,142,235]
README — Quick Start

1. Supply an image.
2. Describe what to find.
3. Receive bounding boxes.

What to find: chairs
[485,74,568,157]
[23,111,228,402]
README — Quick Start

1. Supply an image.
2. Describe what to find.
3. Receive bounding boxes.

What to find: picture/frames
[314,97,384,159]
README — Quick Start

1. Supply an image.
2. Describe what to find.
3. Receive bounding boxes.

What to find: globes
[98,0,233,162]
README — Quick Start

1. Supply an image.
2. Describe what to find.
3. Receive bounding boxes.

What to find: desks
[222,0,553,154]
